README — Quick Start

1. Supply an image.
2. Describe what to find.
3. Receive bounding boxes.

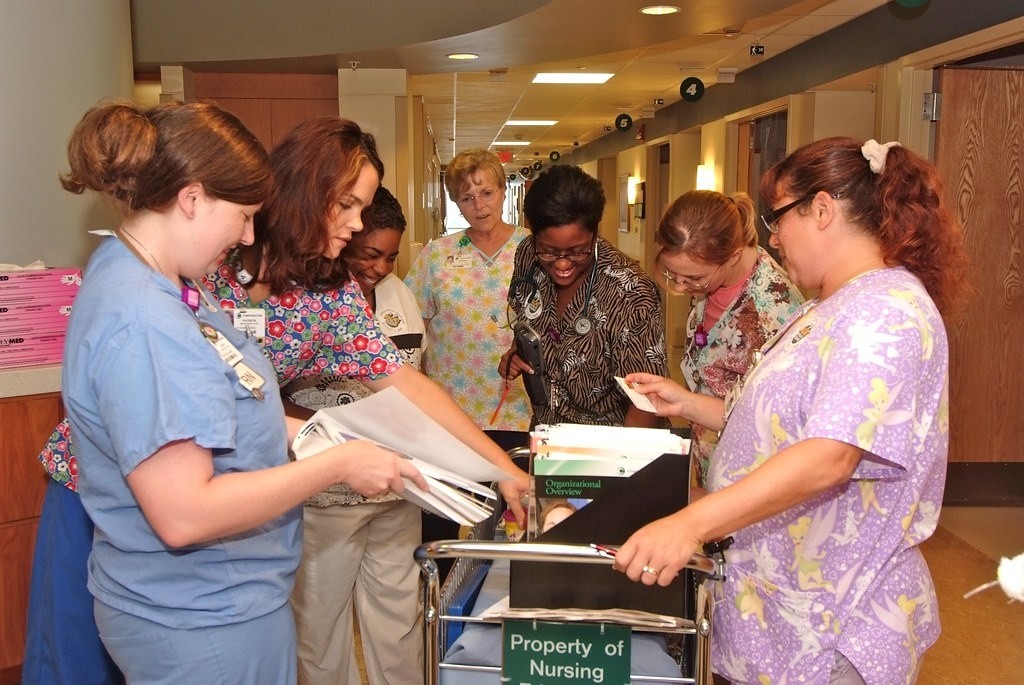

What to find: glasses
[761,193,845,234]
[456,185,499,207]
[661,266,722,293]
[533,234,594,262]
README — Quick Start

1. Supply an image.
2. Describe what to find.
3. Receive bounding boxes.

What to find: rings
[643,565,657,574]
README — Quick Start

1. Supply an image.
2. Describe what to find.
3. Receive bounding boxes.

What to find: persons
[279,185,429,685]
[57,95,429,684]
[401,147,537,629]
[497,162,672,448]
[19,117,567,684]
[612,135,980,685]
[652,186,810,489]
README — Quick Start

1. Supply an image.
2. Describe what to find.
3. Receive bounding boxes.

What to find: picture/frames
[618,173,630,232]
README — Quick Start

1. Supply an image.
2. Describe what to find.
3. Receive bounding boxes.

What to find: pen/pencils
[589,541,616,557]
[490,385,510,427]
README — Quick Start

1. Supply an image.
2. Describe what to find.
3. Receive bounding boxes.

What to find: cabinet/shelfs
[0,393,66,685]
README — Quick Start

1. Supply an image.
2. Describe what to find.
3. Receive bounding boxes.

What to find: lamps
[696,164,709,190]
[626,177,639,205]
